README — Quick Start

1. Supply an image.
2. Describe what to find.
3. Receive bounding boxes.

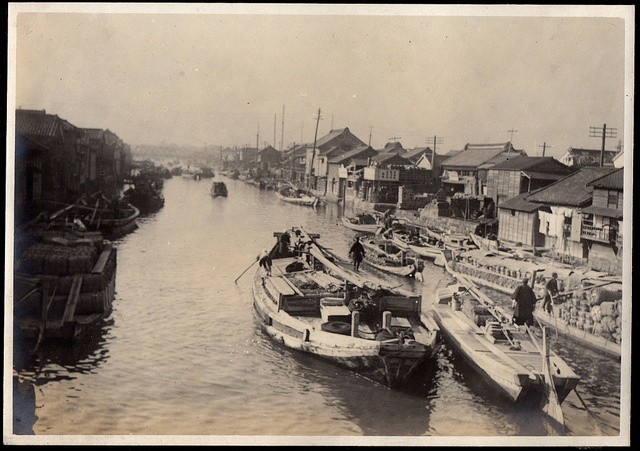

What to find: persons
[256,249,272,277]
[511,278,536,327]
[349,236,366,272]
[543,273,560,317]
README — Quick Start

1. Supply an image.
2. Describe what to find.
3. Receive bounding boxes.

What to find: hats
[521,276,528,282]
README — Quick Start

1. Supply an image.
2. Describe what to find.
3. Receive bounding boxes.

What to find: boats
[123,183,164,209]
[356,229,425,277]
[276,186,319,207]
[382,223,444,257]
[14,223,116,351]
[102,222,139,241]
[252,226,440,391]
[211,180,227,197]
[342,215,385,235]
[432,262,581,425]
[84,201,140,228]
[512,299,621,361]
[14,375,36,433]
